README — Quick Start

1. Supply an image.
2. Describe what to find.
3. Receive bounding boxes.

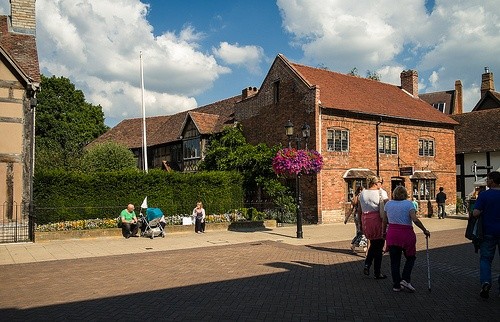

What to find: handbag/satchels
[182,216,193,226]
[379,190,386,219]
[118,220,123,228]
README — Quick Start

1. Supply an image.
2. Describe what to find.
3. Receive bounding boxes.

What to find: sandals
[393,280,415,291]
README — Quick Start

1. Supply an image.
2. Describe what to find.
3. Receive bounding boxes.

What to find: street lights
[284,119,312,238]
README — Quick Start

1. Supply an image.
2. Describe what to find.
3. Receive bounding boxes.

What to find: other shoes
[197,230,205,234]
[363,264,370,275]
[131,233,139,237]
[351,246,358,254]
[373,273,387,280]
[480,281,490,299]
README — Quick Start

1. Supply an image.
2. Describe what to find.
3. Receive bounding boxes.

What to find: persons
[382,186,430,292]
[160,215,167,230]
[344,187,366,253]
[365,189,389,258]
[358,176,385,279]
[412,195,418,213]
[435,187,447,219]
[193,201,205,234]
[119,204,139,239]
[473,171,500,298]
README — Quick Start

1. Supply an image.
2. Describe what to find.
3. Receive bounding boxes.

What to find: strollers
[139,207,167,240]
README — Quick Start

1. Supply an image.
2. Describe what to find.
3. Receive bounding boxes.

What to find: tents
[474,169,500,192]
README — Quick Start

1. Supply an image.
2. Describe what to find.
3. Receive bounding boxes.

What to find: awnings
[409,171,438,180]
[342,168,375,179]
